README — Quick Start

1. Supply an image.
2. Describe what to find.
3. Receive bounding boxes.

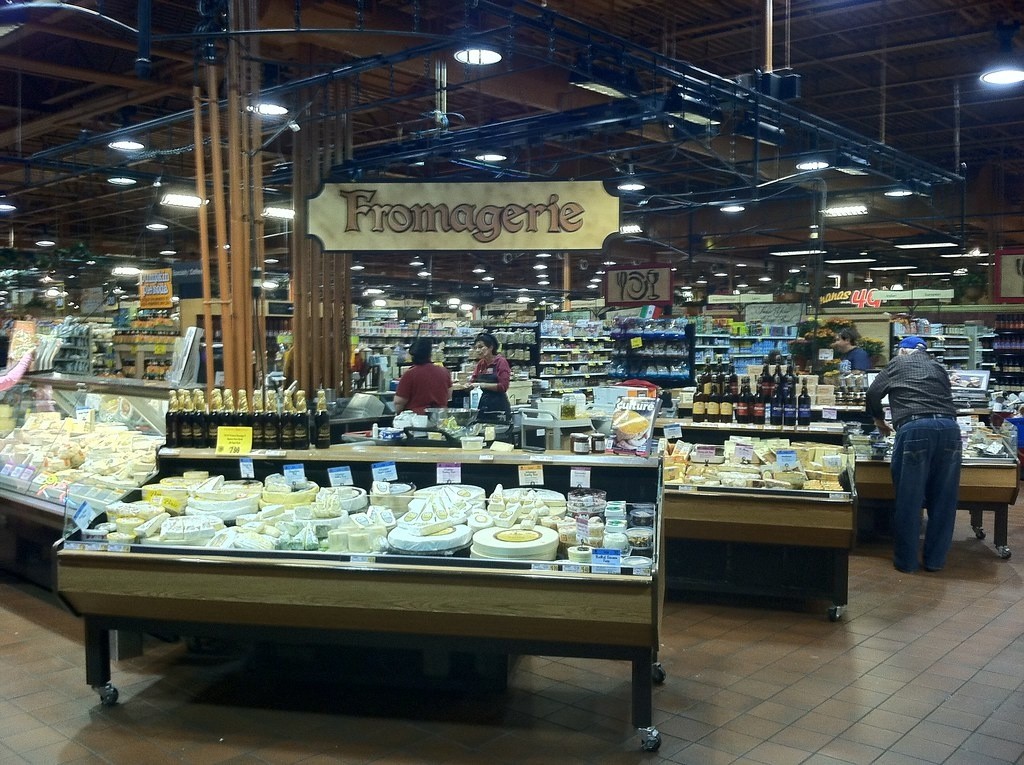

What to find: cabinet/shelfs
[889,319,983,370]
[31,320,797,391]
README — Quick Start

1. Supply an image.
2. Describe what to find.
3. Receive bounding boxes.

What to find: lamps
[474,145,508,162]
[980,20,1024,86]
[451,15,503,65]
[719,194,746,212]
[105,88,289,232]
[616,172,646,192]
[795,148,830,172]
[884,179,912,197]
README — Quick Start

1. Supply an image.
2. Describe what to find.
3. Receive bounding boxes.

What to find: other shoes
[894,564,942,574]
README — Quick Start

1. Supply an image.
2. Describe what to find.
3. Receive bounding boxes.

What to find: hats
[474,333,499,349]
[892,336,928,355]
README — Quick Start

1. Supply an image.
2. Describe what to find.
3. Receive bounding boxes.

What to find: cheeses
[0,404,161,490]
[665,440,853,492]
[88,472,605,563]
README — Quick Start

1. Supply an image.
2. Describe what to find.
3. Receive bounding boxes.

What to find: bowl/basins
[630,508,656,525]
[425,408,480,426]
[460,436,484,450]
[626,528,653,547]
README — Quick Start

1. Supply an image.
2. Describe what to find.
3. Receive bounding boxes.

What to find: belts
[896,413,956,431]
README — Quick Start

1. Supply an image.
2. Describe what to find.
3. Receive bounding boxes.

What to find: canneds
[569,433,605,455]
[833,392,867,406]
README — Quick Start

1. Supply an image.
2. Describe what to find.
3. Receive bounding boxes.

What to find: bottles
[561,394,576,420]
[992,313,1024,386]
[570,432,606,454]
[166,389,330,449]
[941,323,965,336]
[693,353,811,426]
[573,390,586,417]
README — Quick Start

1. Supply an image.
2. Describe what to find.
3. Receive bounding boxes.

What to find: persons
[835,327,872,375]
[393,341,454,417]
[464,334,512,424]
[866,337,962,572]
[950,375,981,388]
[0,348,36,392]
